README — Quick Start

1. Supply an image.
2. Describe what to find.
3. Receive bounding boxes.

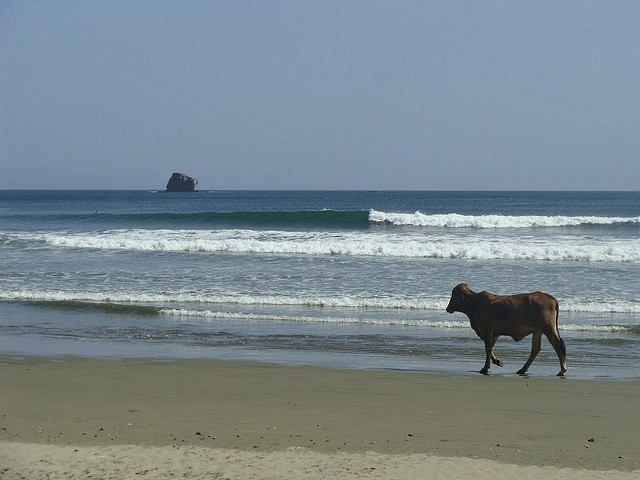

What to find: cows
[446,281,570,378]
